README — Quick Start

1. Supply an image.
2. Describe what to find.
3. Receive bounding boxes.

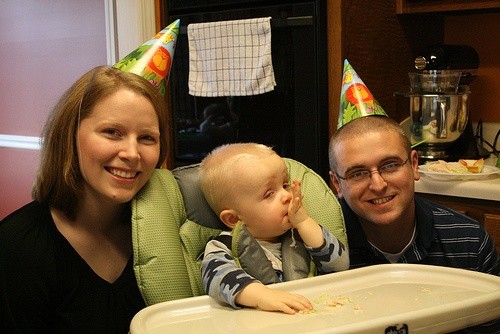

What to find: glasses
[334,154,410,188]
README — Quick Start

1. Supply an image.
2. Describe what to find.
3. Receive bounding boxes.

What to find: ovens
[165,0,330,188]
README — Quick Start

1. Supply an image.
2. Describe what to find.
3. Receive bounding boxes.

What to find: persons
[200,142,350,315]
[0,65,169,334]
[329,114,500,334]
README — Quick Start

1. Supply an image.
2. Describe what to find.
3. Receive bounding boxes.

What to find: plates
[417,164,500,181]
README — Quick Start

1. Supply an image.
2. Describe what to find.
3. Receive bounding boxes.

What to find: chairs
[127,162,500,334]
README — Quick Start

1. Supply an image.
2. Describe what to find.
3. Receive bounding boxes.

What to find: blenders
[392,41,491,165]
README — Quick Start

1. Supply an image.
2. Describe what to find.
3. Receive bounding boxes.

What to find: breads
[458,158,484,173]
